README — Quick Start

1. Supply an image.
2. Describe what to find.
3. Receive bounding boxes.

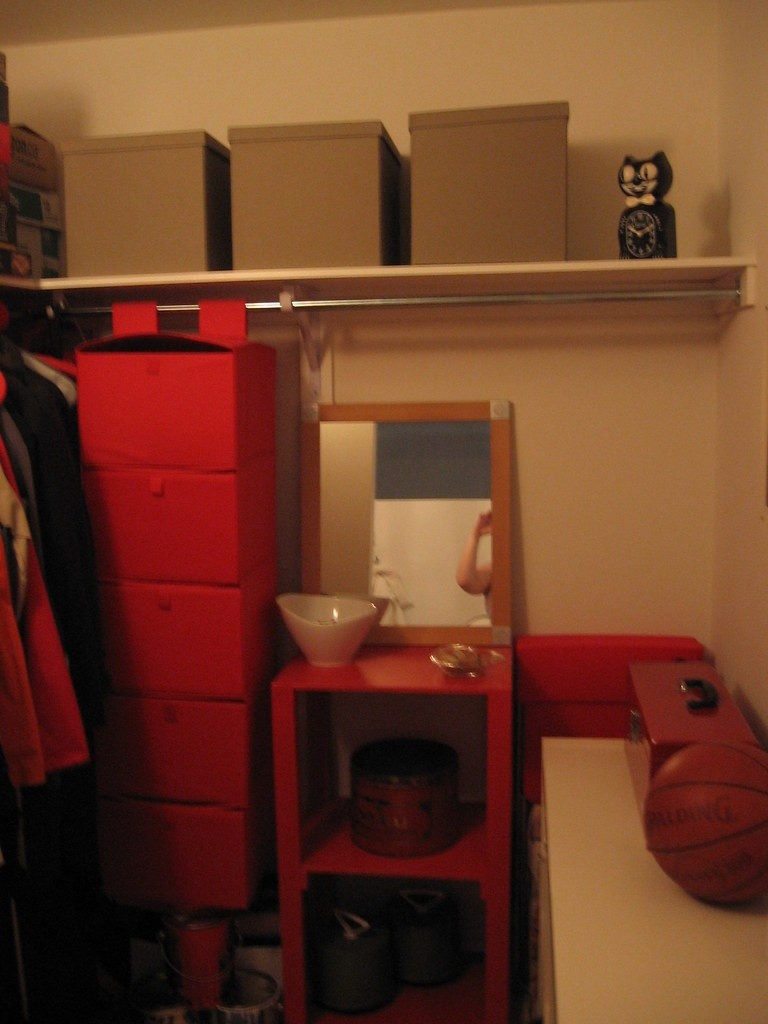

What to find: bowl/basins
[429,644,496,681]
[276,595,378,667]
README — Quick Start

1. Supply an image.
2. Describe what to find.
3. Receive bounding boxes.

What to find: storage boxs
[59,129,234,275]
[76,302,279,912]
[6,124,56,188]
[408,100,571,263]
[0,181,61,278]
[225,118,402,268]
[623,662,764,817]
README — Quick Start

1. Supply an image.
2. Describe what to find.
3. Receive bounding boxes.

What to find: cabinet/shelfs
[271,649,514,1024]
[540,735,768,1024]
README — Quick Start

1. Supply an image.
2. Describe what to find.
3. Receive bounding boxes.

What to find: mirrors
[299,401,511,649]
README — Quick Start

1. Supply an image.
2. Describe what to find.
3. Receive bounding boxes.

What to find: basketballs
[641,739,768,903]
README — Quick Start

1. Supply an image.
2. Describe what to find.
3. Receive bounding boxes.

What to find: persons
[457,509,491,616]
